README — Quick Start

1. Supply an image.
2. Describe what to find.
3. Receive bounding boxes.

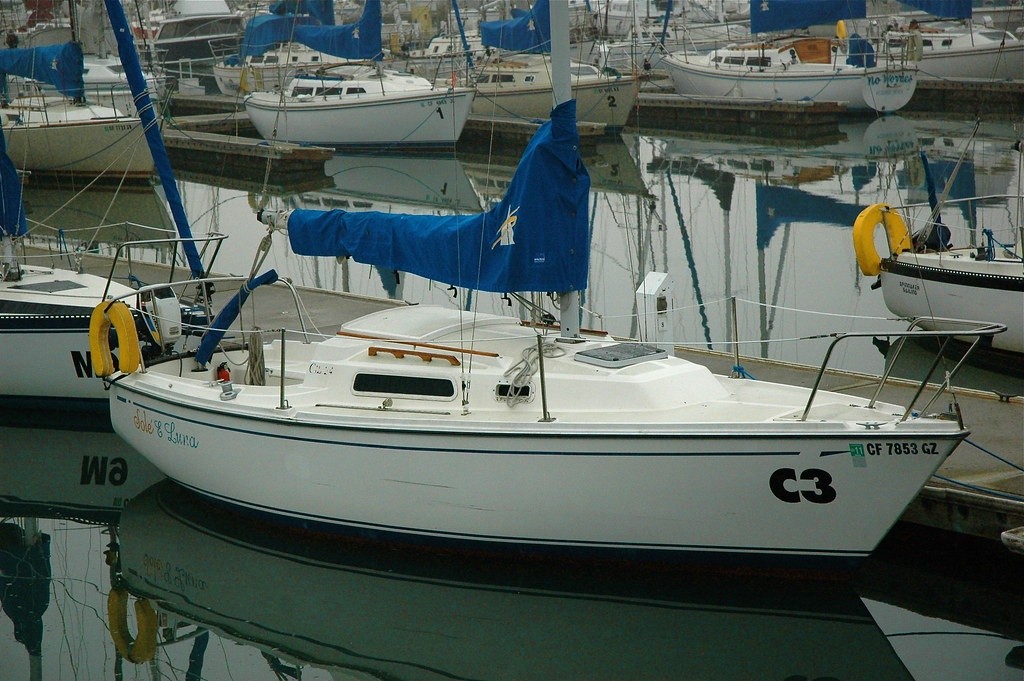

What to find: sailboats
[108,476,915,680]
[0,410,166,680]
[89,1,1010,584]
[0,0,1024,409]
